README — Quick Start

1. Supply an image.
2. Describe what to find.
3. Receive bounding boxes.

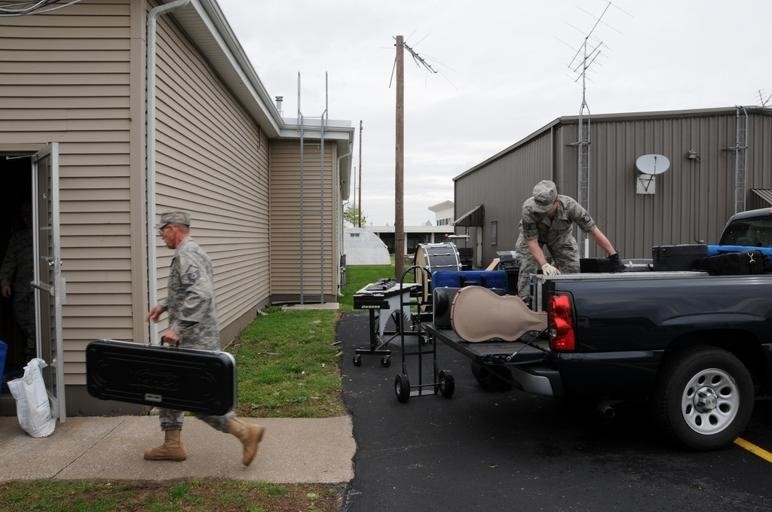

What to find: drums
[414,243,462,283]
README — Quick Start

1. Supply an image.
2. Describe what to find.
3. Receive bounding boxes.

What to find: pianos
[353,279,423,310]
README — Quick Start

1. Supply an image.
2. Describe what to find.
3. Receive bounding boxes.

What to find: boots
[224,416,265,466]
[144,430,188,461]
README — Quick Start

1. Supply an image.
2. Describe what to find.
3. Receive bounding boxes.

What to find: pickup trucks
[425,207,772,449]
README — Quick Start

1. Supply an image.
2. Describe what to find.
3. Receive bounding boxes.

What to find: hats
[532,180,557,213]
[155,212,190,229]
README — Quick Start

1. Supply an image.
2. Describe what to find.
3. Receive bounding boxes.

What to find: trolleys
[352,279,423,368]
[395,265,455,402]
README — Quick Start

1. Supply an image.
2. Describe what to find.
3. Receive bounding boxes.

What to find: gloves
[608,254,624,273]
[541,263,561,276]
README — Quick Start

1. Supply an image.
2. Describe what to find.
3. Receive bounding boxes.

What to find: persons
[142,208,266,466]
[1,199,37,376]
[513,179,627,310]
